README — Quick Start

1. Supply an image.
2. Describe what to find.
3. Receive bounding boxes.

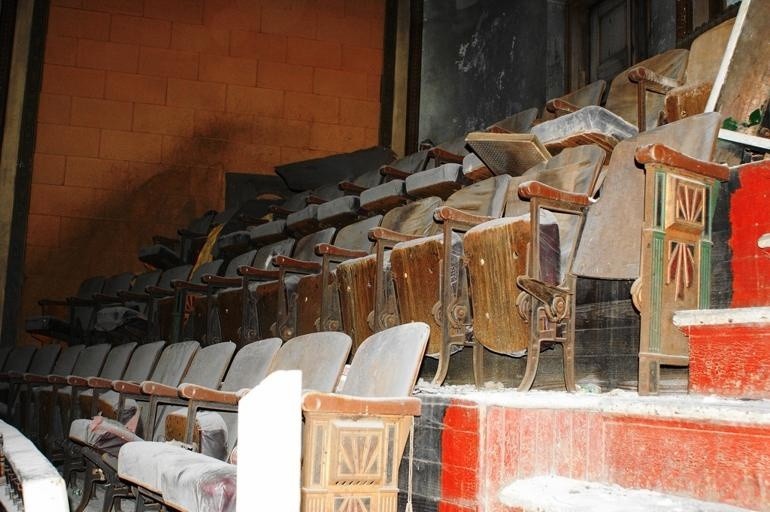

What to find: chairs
[3,320,431,512]
[33,20,736,395]
